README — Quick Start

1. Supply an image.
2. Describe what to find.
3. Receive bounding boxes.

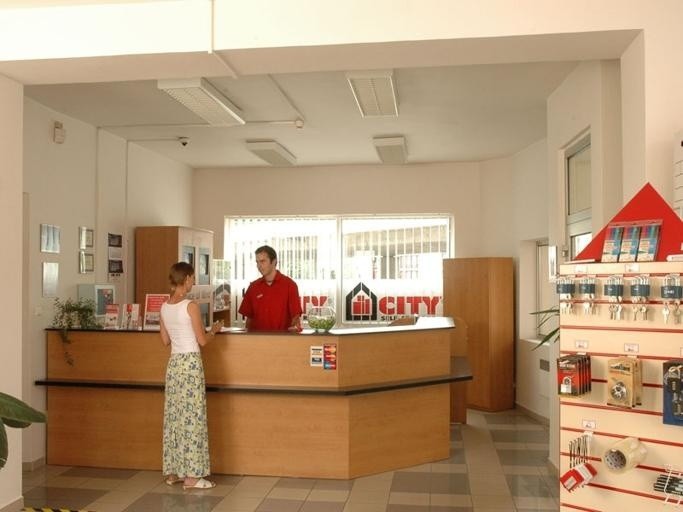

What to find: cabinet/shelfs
[134,225,215,333]
[443,256,516,412]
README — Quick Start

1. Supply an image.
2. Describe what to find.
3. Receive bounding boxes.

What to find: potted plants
[51,296,104,367]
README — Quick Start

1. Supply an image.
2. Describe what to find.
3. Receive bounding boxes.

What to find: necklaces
[262,279,273,284]
[173,293,186,299]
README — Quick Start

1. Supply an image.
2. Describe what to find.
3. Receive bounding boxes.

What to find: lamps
[373,137,407,165]
[246,142,296,166]
[346,69,400,118]
[157,78,246,128]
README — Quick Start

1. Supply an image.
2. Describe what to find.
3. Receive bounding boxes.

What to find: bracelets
[208,330,215,338]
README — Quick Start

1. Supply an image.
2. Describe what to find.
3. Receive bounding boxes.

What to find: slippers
[165,474,217,490]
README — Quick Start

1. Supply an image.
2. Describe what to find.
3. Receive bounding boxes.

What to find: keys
[560,300,683,326]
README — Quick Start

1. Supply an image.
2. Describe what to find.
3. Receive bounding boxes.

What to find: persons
[157,261,226,491]
[237,245,302,332]
